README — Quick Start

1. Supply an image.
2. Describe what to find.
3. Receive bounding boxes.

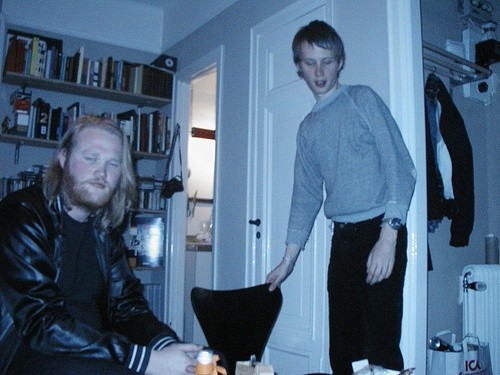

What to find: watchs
[381,216,404,233]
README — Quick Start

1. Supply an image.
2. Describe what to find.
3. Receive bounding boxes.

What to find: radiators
[461,264,500,375]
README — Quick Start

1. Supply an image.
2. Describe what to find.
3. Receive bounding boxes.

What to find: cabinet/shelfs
[0,71,171,269]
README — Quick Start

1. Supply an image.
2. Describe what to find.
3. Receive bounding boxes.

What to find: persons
[0,115,229,375]
[266,18,418,374]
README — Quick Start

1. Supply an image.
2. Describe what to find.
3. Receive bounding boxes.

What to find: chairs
[190,284,283,375]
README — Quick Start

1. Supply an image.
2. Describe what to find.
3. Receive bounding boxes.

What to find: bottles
[427,337,454,351]
[485,234,498,265]
[193,349,214,375]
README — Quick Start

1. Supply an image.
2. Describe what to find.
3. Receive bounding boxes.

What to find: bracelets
[282,256,295,266]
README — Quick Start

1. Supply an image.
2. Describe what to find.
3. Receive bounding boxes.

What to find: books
[0,34,176,209]
